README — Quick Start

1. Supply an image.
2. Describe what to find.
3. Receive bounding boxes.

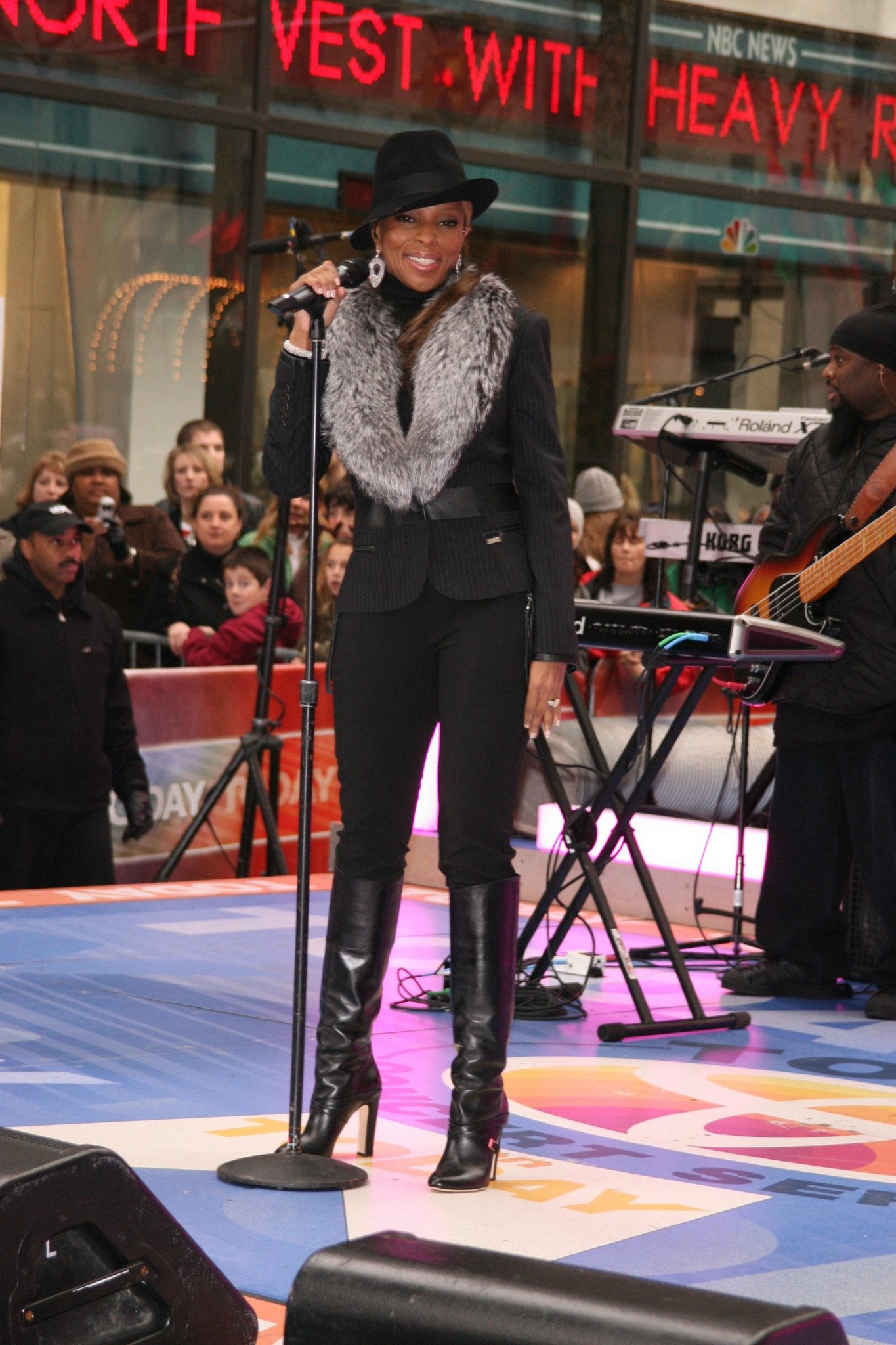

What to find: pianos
[518,600,846,1043]
[614,401,832,826]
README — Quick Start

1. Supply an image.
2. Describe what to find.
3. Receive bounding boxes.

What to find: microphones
[245,230,354,256]
[791,353,831,371]
[267,258,371,316]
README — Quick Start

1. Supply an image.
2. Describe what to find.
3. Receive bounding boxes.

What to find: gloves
[120,790,156,844]
[102,515,130,562]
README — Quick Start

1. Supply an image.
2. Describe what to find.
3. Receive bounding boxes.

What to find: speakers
[0,1128,259,1344]
[285,1231,849,1345]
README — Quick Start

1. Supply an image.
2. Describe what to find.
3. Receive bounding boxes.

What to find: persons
[0,308,896,1020]
[261,131,583,1188]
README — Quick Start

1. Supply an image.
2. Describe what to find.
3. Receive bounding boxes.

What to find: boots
[274,871,404,1159]
[426,871,522,1191]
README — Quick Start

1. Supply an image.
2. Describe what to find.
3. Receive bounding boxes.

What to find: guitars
[733,505,896,706]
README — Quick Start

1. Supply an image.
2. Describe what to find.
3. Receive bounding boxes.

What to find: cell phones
[95,495,116,528]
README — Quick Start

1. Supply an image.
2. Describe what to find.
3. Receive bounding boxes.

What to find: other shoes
[719,956,838,998]
[864,989,896,1021]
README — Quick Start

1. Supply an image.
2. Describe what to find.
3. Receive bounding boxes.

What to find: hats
[830,305,896,371]
[573,467,623,514]
[15,505,95,536]
[63,438,128,477]
[349,128,500,253]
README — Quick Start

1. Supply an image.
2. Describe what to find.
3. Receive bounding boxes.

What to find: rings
[548,698,560,709]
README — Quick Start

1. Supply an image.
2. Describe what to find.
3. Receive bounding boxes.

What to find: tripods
[152,495,288,883]
